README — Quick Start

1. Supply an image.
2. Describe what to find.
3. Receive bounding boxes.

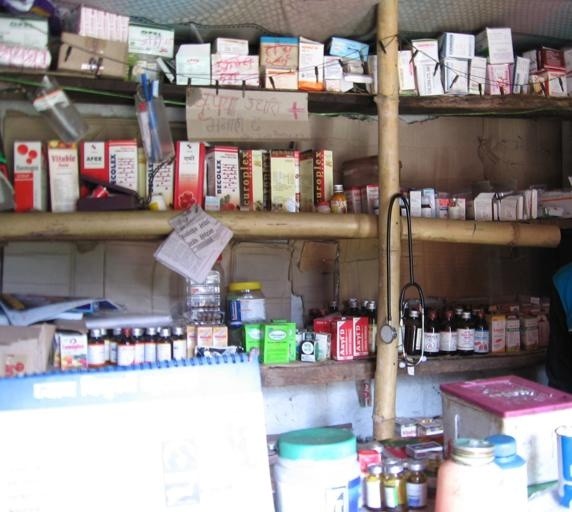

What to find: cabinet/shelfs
[0,0,572,380]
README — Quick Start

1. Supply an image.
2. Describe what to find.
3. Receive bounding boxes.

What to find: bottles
[227,281,267,352]
[274,416,572,512]
[88,324,186,368]
[372,196,460,220]
[401,299,488,359]
[329,297,376,354]
[342,154,402,188]
[317,183,348,214]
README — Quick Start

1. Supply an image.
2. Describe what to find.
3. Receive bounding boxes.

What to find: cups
[0,152,15,212]
[135,93,175,163]
[33,78,90,145]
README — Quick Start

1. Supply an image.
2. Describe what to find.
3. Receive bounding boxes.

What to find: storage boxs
[434,373,572,489]
